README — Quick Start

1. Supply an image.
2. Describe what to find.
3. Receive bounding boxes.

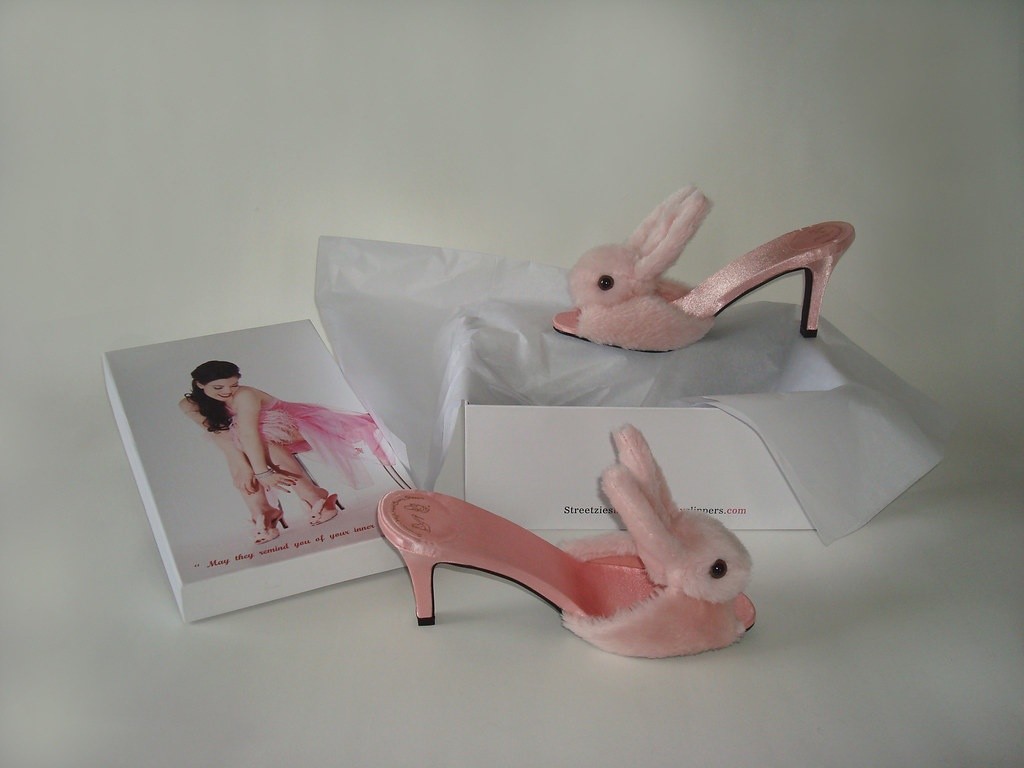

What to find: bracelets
[254,469,273,479]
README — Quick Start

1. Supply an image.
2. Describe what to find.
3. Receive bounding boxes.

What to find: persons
[180,360,395,544]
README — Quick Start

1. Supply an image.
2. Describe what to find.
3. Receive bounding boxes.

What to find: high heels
[552,187,855,354]
[251,506,288,545]
[377,423,756,659]
[300,490,345,526]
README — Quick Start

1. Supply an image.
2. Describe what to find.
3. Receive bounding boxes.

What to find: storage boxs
[459,315,832,531]
[104,317,416,627]
